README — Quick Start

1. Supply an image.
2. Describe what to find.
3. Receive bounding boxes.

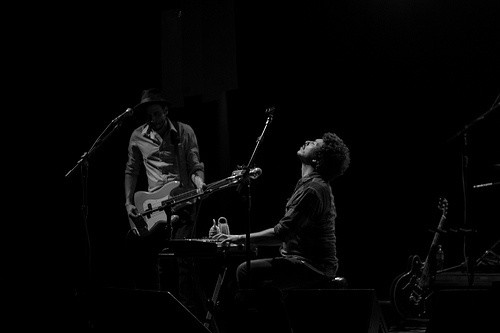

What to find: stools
[281,276,346,333]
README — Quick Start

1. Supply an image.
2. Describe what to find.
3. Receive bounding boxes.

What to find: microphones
[112,108,132,123]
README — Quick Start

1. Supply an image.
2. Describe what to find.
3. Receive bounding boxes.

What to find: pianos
[171,238,246,255]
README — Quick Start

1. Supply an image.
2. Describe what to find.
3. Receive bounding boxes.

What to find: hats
[134,89,172,110]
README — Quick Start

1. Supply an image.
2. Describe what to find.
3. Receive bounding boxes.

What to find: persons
[216,131,351,333]
[124,87,205,240]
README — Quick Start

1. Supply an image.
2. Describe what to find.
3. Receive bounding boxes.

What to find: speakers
[285,288,389,333]
[88,288,211,333]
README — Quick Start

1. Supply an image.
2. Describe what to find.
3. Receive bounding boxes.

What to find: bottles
[435,244,444,272]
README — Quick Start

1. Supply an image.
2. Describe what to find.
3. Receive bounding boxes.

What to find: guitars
[127,164,263,242]
[389,197,449,319]
